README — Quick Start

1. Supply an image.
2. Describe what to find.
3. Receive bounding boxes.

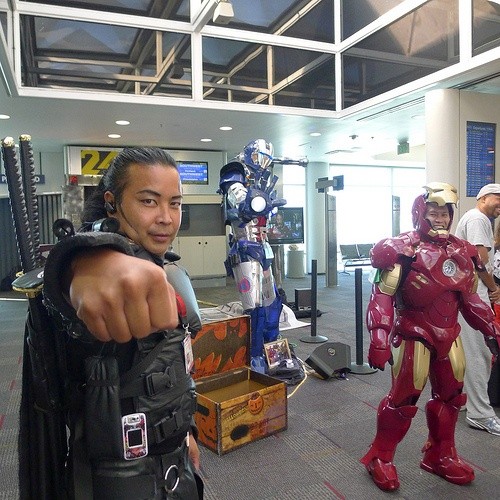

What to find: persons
[268,213,291,239]
[20,147,210,500]
[220,139,287,372]
[360,183,500,489]
[455,183,500,435]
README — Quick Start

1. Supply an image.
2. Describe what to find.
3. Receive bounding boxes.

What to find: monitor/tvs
[175,160,209,185]
[267,206,305,245]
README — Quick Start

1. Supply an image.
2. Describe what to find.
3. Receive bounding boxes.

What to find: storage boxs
[191,315,288,456]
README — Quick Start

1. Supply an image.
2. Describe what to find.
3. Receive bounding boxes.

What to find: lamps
[398,137,409,155]
[213,0,234,25]
[169,59,184,79]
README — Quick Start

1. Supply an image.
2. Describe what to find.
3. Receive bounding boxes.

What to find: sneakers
[466,415,500,436]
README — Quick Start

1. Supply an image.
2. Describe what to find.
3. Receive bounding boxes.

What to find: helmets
[243,139,274,173]
[411,182,459,244]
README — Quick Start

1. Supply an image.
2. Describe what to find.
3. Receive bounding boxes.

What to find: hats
[475,184,500,201]
